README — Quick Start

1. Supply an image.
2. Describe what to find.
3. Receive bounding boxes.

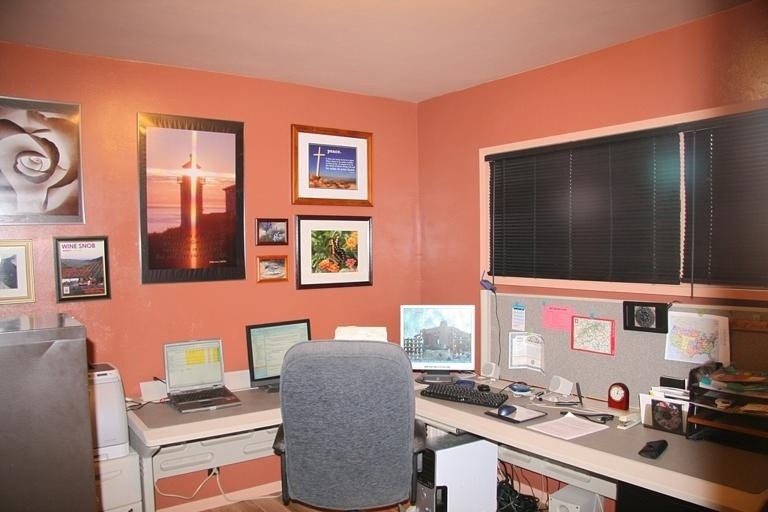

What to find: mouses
[478,384,490,391]
[498,405,517,417]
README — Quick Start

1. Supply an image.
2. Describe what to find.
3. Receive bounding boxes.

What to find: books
[639,365,767,436]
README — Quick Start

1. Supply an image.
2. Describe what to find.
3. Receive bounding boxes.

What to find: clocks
[607,381,630,410]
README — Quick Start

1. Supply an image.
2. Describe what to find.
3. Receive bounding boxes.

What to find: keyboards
[421,384,508,408]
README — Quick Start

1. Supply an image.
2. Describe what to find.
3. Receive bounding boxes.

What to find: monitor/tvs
[400,305,475,384]
[245,318,312,393]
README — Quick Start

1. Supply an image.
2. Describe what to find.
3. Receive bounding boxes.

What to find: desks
[127,372,480,512]
[413,376,768,512]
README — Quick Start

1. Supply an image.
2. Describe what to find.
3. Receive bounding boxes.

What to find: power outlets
[207,466,221,477]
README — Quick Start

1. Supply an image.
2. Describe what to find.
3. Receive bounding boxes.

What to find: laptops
[162,339,243,414]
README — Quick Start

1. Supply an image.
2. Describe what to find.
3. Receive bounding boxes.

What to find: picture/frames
[52,236,111,303]
[0,95,88,226]
[256,255,288,283]
[291,124,374,208]
[135,112,245,283]
[295,214,373,289]
[256,217,288,246]
[1,239,36,306]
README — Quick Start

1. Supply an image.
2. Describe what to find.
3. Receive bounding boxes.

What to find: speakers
[481,361,501,382]
[549,375,574,398]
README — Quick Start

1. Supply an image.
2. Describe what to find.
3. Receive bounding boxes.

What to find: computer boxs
[416,433,498,512]
[548,485,603,512]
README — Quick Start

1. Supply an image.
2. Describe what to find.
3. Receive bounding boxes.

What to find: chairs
[271,340,436,512]
